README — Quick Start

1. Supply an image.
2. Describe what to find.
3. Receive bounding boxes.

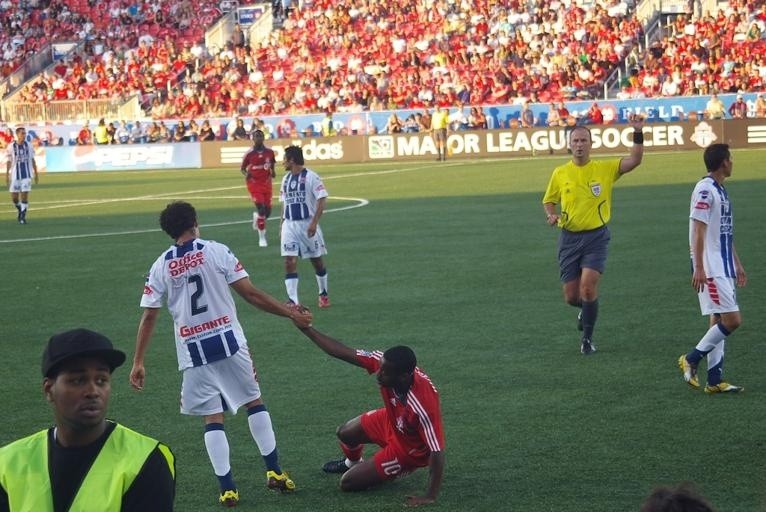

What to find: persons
[130,202,295,505]
[677,145,745,395]
[279,146,328,309]
[131,121,145,141]
[706,94,724,120]
[409,114,425,132]
[421,110,432,132]
[5,129,12,148]
[241,130,275,248]
[729,96,747,119]
[95,119,108,145]
[633,487,714,512]
[148,123,159,142]
[174,122,187,142]
[547,104,560,127]
[189,121,199,134]
[252,120,270,140]
[158,122,171,142]
[323,102,334,137]
[445,110,449,117]
[470,108,481,129]
[6,128,38,223]
[544,115,645,356]
[287,304,444,506]
[78,126,91,144]
[107,124,116,145]
[226,115,237,141]
[238,120,245,139]
[0,329,176,511]
[590,103,603,126]
[618,0,766,99]
[387,115,401,134]
[755,95,766,117]
[431,104,447,161]
[115,120,129,144]
[199,121,215,142]
[521,104,533,128]
[384,0,618,110]
[0,0,387,120]
[478,108,487,130]
[557,102,570,120]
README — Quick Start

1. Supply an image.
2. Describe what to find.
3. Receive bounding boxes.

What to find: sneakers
[323,457,349,473]
[318,294,329,308]
[582,337,594,353]
[18,212,20,222]
[19,216,25,224]
[253,213,259,230]
[679,353,701,387]
[219,489,239,507]
[259,237,267,247]
[266,469,295,494]
[577,310,584,331]
[704,380,744,393]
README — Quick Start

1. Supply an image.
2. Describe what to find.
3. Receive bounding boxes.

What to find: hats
[41,329,125,377]
